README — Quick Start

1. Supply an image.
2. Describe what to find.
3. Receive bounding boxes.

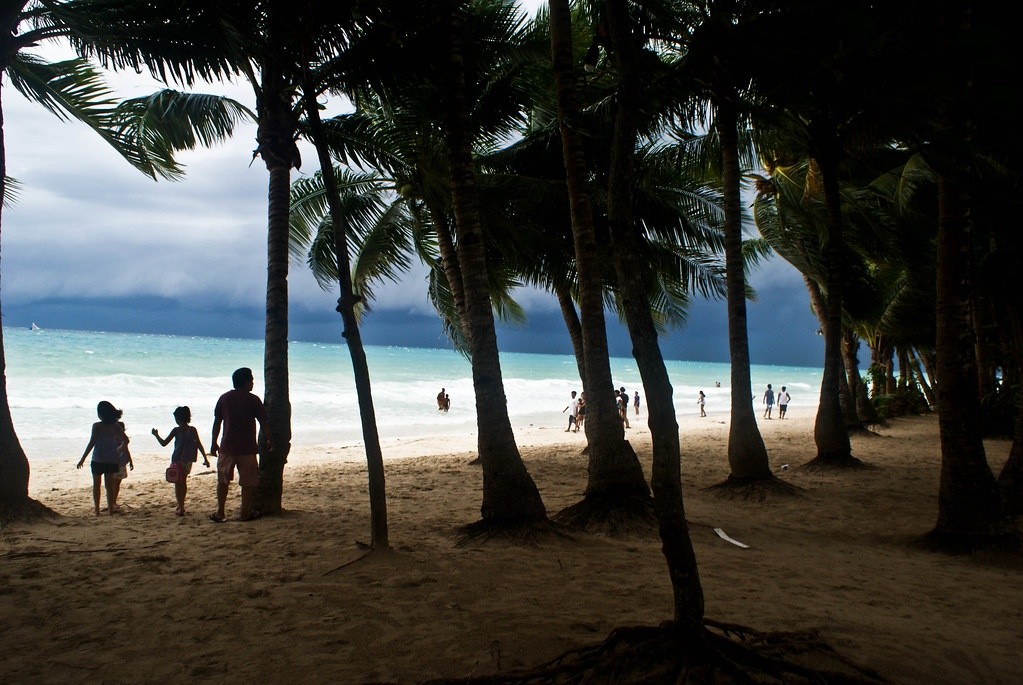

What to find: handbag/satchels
[166,463,182,483]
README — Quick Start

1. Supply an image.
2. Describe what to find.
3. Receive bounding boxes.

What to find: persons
[151,405,210,516]
[209,367,273,522]
[563,391,585,433]
[614,388,630,428]
[76,401,129,515]
[698,390,707,417]
[113,422,134,508]
[715,381,720,387]
[763,384,775,419]
[437,387,450,412]
[777,386,791,419]
[634,391,640,415]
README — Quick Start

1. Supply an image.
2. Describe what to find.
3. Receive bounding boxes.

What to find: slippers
[240,511,259,521]
[210,514,227,522]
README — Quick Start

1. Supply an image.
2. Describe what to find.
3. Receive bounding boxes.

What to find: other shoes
[109,505,124,515]
[175,506,185,516]
[92,509,99,514]
